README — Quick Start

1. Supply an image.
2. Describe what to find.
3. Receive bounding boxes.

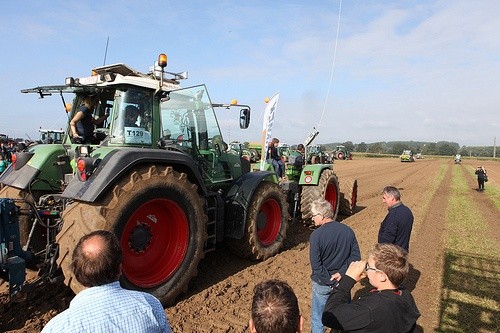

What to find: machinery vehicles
[301,144,334,164]
[0,52,291,310]
[400,150,414,162]
[237,143,358,229]
[37,127,66,146]
[332,146,353,159]
[228,141,259,162]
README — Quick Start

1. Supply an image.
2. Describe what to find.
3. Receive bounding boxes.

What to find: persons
[108,105,140,129]
[322,243,421,333]
[289,144,304,172]
[69,95,109,144]
[0,140,30,175]
[475,166,486,192]
[249,278,304,333]
[266,138,286,182]
[378,186,414,255]
[40,230,173,333]
[310,198,361,333]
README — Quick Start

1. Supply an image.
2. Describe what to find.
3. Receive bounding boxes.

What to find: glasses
[309,213,325,218]
[365,263,388,280]
